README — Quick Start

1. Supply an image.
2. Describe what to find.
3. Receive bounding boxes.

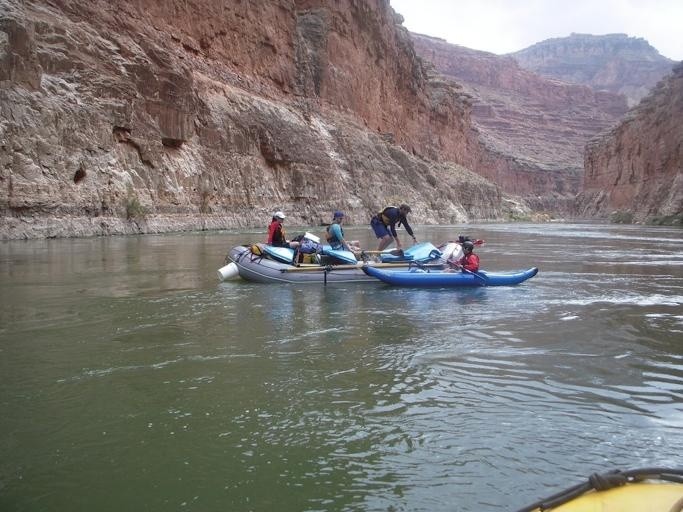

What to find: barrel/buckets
[217,262,239,281]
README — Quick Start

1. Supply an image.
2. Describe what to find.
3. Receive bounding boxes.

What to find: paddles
[353,249,403,257]
[429,250,488,286]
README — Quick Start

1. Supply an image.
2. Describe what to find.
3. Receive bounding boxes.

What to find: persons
[267,212,300,249]
[325,211,361,262]
[428,241,479,274]
[369,203,418,259]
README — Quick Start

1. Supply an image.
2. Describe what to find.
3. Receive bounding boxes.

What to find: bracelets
[413,237,416,239]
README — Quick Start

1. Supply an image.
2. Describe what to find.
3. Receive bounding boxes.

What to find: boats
[362,264,539,287]
[216,235,486,283]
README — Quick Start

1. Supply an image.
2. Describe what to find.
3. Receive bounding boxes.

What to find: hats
[460,241,474,249]
[334,212,343,217]
[400,204,412,213]
[275,212,286,219]
